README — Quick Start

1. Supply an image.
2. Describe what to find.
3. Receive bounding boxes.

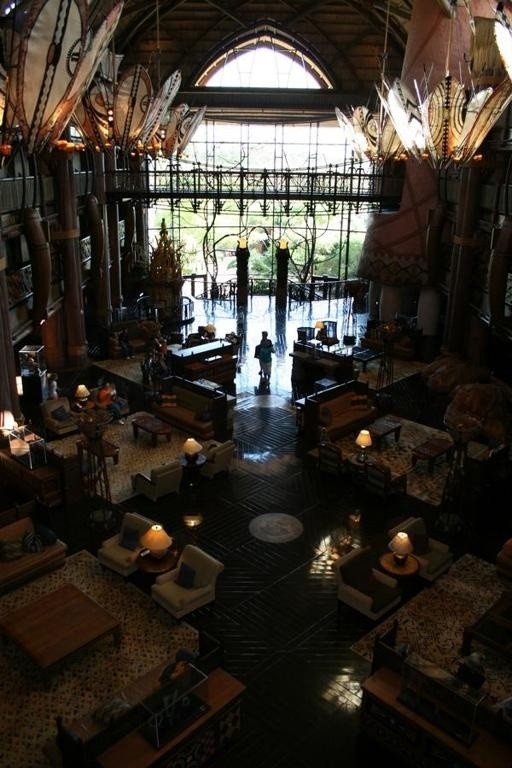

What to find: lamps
[234,236,249,307]
[145,104,206,159]
[388,532,414,566]
[415,68,512,171]
[112,52,181,158]
[0,1,124,157]
[68,50,117,154]
[140,523,172,563]
[355,429,372,462]
[75,383,89,410]
[373,64,441,167]
[492,19,512,82]
[314,320,324,340]
[183,438,203,464]
[335,102,409,171]
[275,239,292,307]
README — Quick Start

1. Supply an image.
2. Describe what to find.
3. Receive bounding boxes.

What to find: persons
[98,379,124,425]
[226,332,237,344]
[235,305,244,335]
[119,327,135,359]
[158,649,191,692]
[260,340,276,380]
[36,364,49,403]
[201,330,209,339]
[254,332,268,374]
[49,373,59,400]
[455,651,486,689]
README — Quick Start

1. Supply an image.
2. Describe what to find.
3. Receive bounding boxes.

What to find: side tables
[378,553,421,578]
[137,555,175,574]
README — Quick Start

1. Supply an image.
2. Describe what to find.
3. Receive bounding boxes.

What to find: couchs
[496,538,512,577]
[150,375,227,444]
[293,340,360,378]
[197,439,236,481]
[41,396,81,440]
[112,331,161,359]
[388,517,454,585]
[365,462,408,501]
[91,385,129,422]
[151,544,224,621]
[317,443,349,479]
[97,512,163,584]
[363,326,423,360]
[130,461,184,503]
[0,516,68,592]
[335,546,402,631]
[374,618,512,748]
[304,379,380,444]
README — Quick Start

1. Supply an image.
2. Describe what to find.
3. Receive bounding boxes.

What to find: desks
[296,395,306,432]
[96,667,248,767]
[185,354,233,380]
[172,339,233,376]
[360,668,512,767]
[225,393,237,437]
[288,350,338,369]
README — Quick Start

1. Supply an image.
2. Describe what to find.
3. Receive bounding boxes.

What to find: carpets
[324,413,491,509]
[93,354,174,390]
[43,411,206,506]
[349,552,512,725]
[0,548,201,768]
[331,343,428,389]
[334,345,384,362]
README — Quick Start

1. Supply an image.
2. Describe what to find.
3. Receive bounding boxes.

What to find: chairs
[54,631,226,768]
[297,327,315,342]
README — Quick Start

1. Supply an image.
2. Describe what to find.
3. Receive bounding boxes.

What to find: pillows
[93,696,131,724]
[0,541,25,560]
[118,526,139,550]
[339,564,370,585]
[174,561,196,588]
[195,408,211,422]
[408,533,429,555]
[52,406,71,420]
[160,393,178,407]
[23,530,43,552]
[321,407,333,423]
[350,395,368,410]
